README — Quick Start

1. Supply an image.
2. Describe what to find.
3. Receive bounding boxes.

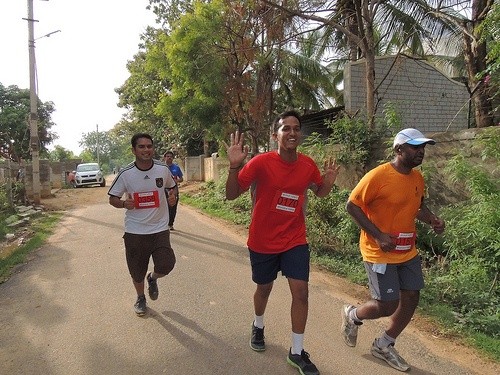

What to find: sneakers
[249,320,266,351]
[341,303,363,347]
[134,295,146,316]
[168,225,174,230]
[147,272,159,300]
[370,337,410,372]
[287,346,320,375]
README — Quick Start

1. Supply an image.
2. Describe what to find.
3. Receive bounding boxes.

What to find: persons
[164,151,183,231]
[340,127,446,372]
[108,132,177,316]
[112,167,116,175]
[66,171,76,187]
[220,110,340,374]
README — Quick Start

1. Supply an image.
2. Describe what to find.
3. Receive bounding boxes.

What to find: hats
[393,127,437,146]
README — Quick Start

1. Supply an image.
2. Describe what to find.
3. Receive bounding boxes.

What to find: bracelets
[122,201,126,208]
[230,166,239,170]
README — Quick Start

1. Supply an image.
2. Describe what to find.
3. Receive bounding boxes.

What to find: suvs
[71,163,106,189]
